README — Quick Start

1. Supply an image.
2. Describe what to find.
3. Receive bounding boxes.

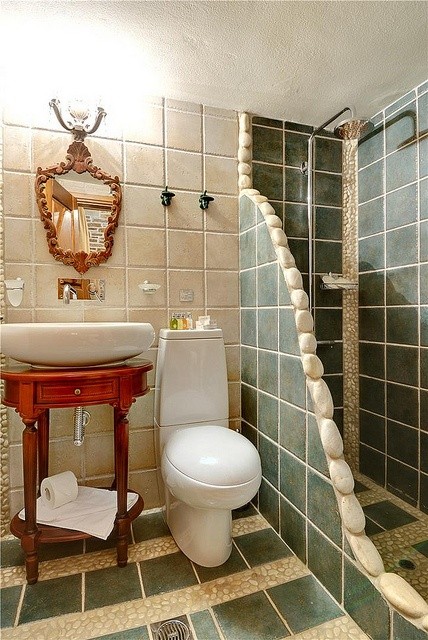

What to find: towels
[17,484,141,541]
[74,205,91,257]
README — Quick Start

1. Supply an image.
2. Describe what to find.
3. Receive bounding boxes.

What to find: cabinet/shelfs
[0,364,153,587]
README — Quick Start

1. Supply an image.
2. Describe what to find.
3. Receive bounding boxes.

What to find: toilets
[153,328,263,569]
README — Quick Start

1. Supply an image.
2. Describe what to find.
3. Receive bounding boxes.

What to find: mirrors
[33,141,123,276]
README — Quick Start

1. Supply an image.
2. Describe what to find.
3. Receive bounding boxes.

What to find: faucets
[58,280,81,311]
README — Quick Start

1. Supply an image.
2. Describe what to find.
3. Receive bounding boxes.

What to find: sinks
[3,323,150,329]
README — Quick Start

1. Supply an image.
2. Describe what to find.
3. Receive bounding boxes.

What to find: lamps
[47,88,109,143]
